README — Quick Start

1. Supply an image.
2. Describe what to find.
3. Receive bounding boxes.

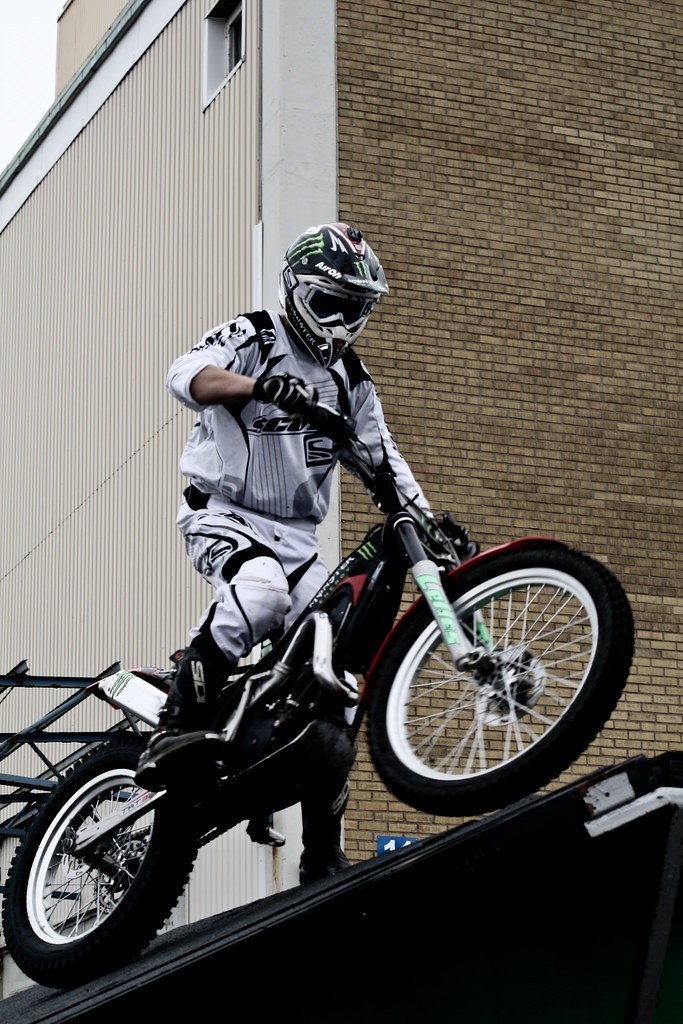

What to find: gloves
[252,370,319,420]
[426,510,470,558]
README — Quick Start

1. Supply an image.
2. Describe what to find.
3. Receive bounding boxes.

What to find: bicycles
[0,371,634,994]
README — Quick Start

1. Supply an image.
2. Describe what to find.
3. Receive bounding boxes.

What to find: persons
[168,222,441,886]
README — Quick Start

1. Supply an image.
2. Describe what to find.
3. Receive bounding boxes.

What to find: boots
[133,648,233,794]
[297,783,352,885]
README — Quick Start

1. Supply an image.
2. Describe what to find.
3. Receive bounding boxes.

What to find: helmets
[278,222,389,368]
